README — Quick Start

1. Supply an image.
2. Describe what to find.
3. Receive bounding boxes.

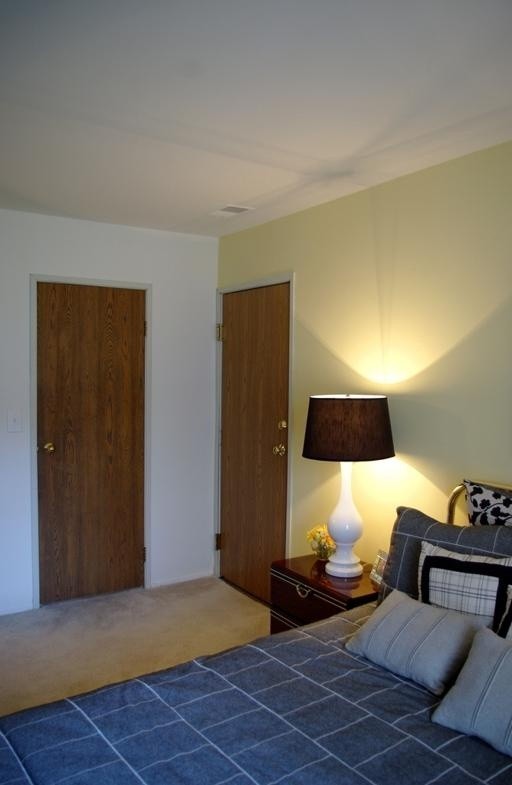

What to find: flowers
[307,526,336,558]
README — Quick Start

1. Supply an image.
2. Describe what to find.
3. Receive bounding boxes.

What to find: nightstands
[270,554,377,634]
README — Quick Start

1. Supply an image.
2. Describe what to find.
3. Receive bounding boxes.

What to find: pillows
[376,506,511,608]
[462,480,512,528]
[416,540,512,633]
[429,628,511,757]
[498,601,511,637]
[342,588,489,695]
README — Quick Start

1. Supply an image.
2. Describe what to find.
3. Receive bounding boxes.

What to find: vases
[313,549,332,562]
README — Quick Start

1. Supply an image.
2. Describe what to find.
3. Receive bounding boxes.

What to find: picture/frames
[369,548,389,584]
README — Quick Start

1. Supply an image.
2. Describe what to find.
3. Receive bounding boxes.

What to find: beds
[0,480,511,785]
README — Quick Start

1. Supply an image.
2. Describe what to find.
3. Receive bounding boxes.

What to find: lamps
[301,394,395,580]
